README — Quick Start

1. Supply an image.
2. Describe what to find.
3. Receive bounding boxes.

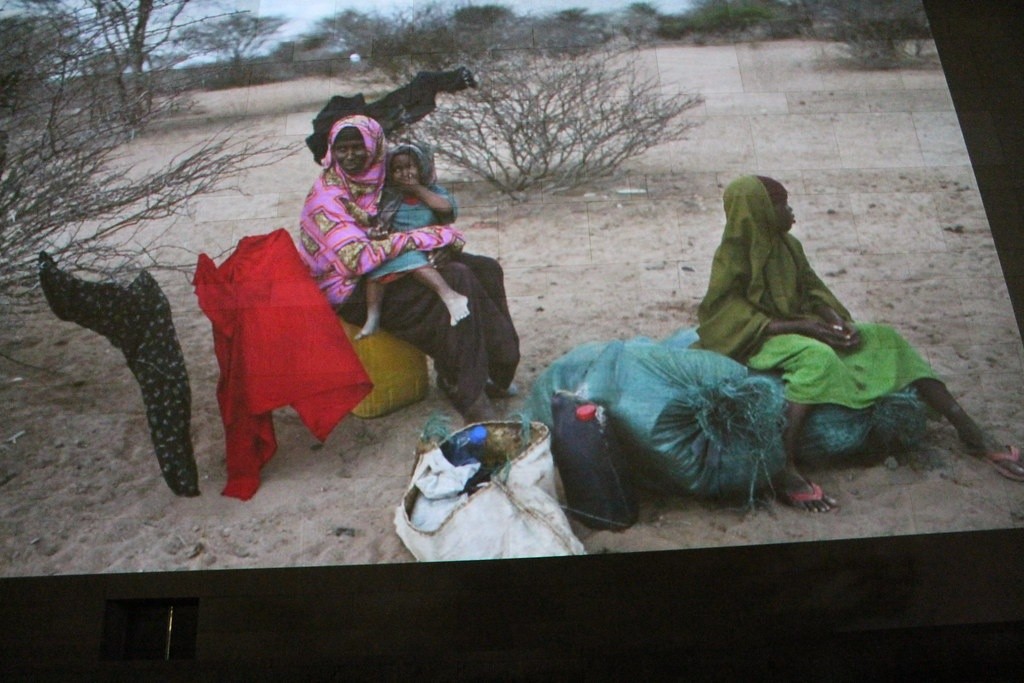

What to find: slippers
[963,437,1024,482]
[770,474,838,514]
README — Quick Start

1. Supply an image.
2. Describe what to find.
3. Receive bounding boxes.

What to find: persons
[687,173,1024,513]
[353,142,471,341]
[299,115,520,427]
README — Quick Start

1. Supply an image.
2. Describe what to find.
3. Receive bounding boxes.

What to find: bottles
[448,426,487,468]
